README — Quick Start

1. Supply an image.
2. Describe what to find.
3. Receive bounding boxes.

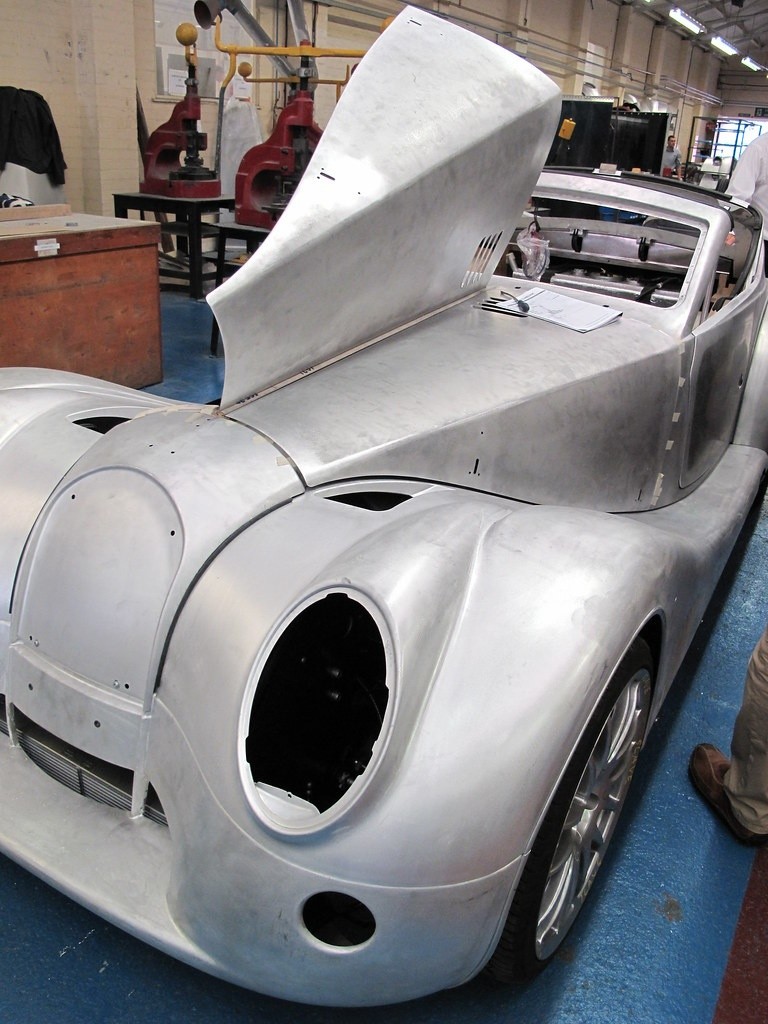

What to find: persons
[686,626,768,849]
[724,130,768,278]
[660,136,683,182]
[623,102,633,112]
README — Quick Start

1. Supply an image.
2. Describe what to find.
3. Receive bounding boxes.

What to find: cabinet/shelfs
[0,211,158,390]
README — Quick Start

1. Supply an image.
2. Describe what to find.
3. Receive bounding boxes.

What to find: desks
[211,220,271,356]
[112,191,236,299]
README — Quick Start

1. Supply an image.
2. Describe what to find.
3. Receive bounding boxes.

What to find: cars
[2,5,768,1017]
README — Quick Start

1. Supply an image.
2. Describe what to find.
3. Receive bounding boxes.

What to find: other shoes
[688,743,756,846]
